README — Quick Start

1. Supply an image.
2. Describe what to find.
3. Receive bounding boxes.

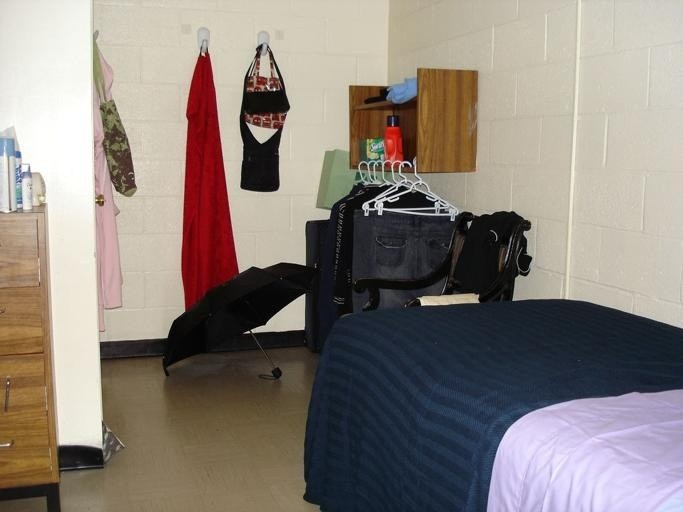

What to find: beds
[302,300,682,511]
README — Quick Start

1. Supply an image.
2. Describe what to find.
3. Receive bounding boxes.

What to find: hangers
[354,158,459,223]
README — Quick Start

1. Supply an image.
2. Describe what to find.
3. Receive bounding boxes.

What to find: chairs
[355,215,527,310]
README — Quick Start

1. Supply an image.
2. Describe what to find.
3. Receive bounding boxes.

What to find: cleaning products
[383,115,404,168]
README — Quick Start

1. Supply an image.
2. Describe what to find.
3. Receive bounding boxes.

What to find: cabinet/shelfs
[349,68,479,172]
[0,202,63,512]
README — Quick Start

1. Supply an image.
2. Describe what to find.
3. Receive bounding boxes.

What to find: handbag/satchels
[240,69,289,192]
[100,95,138,198]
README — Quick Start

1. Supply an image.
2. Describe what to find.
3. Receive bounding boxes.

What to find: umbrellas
[161,262,320,380]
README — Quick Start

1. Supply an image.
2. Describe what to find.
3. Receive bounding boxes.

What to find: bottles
[0,138,33,213]
[384,115,403,168]
